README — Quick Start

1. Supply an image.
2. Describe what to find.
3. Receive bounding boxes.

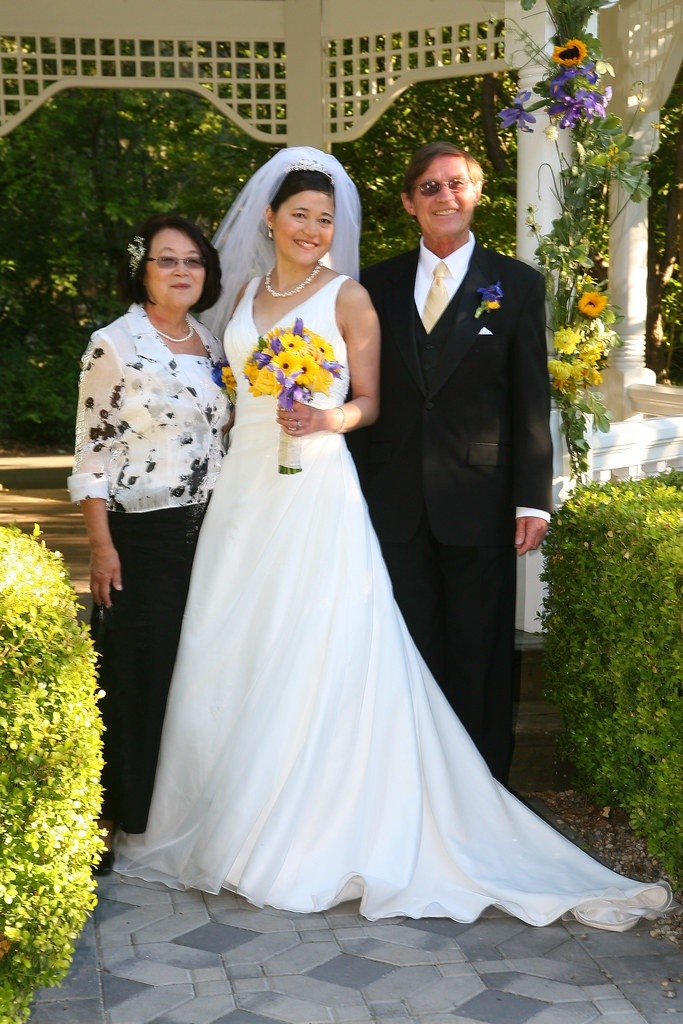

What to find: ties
[421,261,448,335]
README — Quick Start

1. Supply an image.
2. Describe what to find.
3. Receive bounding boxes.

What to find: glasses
[412,178,469,196]
[141,255,204,269]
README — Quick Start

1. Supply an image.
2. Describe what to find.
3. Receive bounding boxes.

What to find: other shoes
[90,822,116,876]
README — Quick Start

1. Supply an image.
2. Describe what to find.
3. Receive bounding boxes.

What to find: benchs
[0,455,93,545]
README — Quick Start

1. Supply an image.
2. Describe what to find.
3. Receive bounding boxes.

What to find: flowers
[127,235,147,278]
[495,0,616,144]
[474,282,504,319]
[241,316,344,475]
[211,356,238,406]
[523,81,683,481]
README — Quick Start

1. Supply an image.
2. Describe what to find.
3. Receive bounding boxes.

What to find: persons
[215,143,383,907]
[69,215,232,875]
[353,141,556,782]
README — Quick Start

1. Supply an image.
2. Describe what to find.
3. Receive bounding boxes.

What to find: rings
[296,419,303,430]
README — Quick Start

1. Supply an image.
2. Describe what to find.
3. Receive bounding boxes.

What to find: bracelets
[334,406,345,434]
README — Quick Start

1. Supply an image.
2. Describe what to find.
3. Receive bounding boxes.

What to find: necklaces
[149,316,194,342]
[264,261,324,297]
